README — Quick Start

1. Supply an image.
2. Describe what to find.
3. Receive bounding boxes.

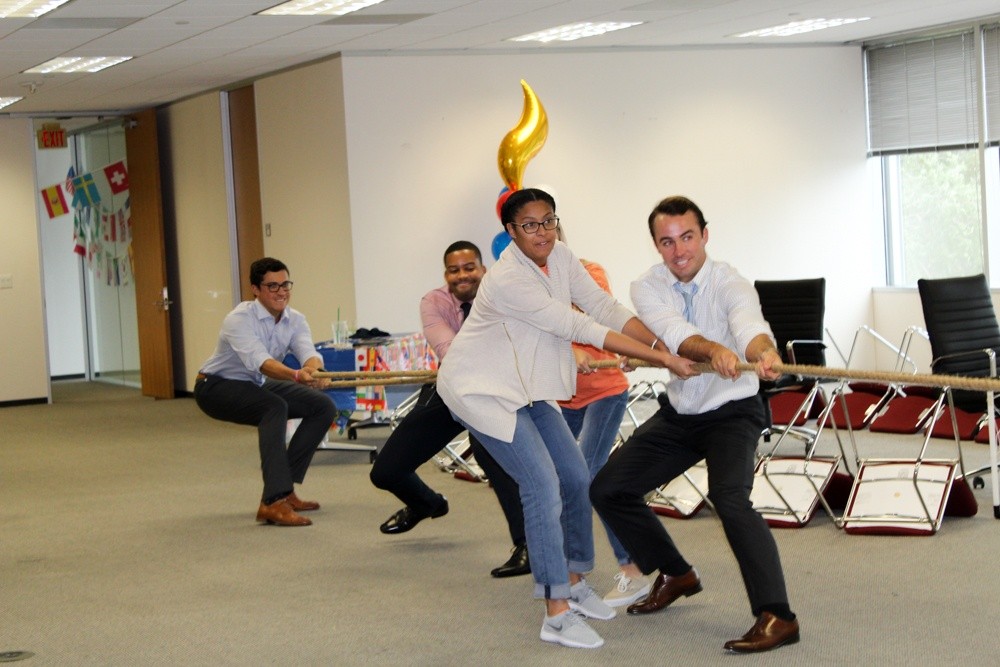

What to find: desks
[281,332,441,439]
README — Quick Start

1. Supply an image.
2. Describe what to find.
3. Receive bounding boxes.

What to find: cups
[332,320,347,352]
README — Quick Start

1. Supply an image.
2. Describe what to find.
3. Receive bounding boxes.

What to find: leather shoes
[724,611,800,654]
[627,565,703,615]
[255,498,311,527]
[285,492,319,511]
[380,494,448,534]
[491,543,532,577]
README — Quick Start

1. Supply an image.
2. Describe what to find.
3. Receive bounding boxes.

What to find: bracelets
[295,370,298,382]
[651,336,659,350]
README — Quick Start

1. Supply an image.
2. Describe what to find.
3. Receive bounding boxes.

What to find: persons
[590,197,801,655]
[194,258,337,526]
[538,226,655,609]
[435,189,700,648]
[370,240,531,578]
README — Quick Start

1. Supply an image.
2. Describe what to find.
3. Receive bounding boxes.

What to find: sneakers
[540,609,604,648]
[567,577,616,619]
[602,571,649,606]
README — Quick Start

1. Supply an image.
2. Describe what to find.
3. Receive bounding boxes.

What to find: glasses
[509,215,560,233]
[260,281,293,293]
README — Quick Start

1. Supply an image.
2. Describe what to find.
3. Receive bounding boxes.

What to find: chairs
[391,388,488,483]
[611,275,1000,536]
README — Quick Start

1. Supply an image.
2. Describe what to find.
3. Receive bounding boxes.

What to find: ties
[459,302,471,321]
[674,281,698,328]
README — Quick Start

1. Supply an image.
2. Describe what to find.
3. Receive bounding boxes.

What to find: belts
[196,374,205,378]
